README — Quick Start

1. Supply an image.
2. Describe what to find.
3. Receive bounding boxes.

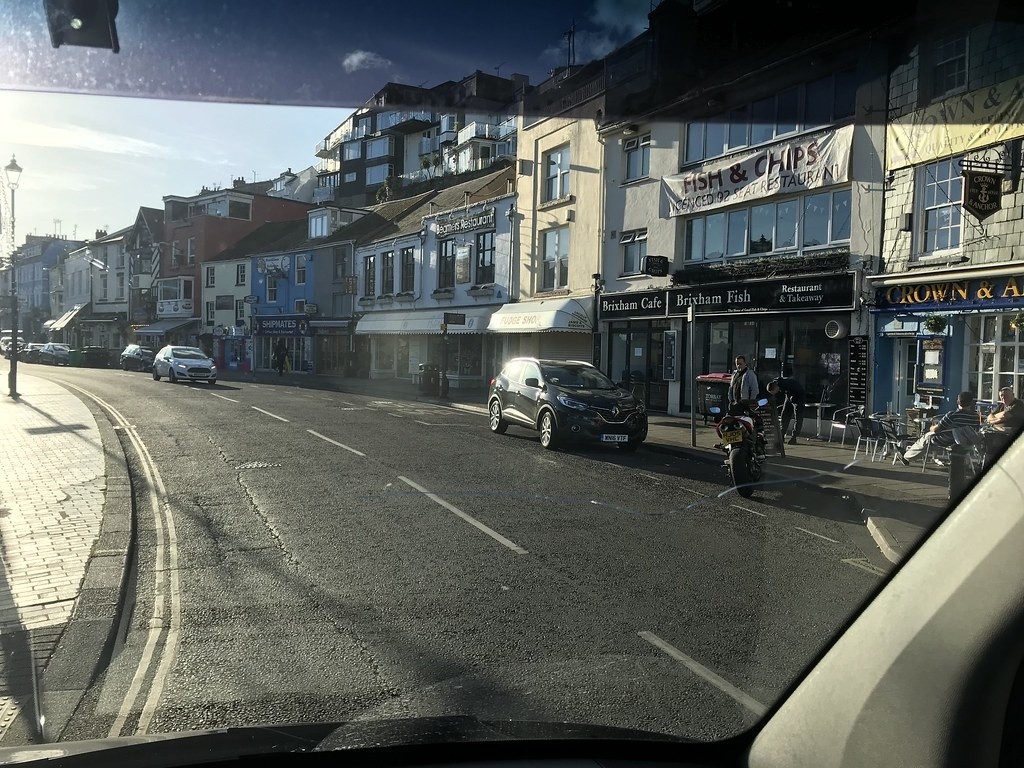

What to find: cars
[20,342,46,363]
[38,342,71,366]
[0,336,26,359]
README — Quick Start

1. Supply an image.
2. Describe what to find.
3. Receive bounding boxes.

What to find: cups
[886,400,894,416]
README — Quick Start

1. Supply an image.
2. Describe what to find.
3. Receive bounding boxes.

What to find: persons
[981,387,1024,472]
[713,356,759,449]
[767,377,805,444]
[893,392,979,466]
[272,340,286,375]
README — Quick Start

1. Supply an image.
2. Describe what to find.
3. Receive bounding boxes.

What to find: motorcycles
[709,398,768,500]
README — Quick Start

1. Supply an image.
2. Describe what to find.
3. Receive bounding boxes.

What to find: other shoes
[896,453,909,466]
[788,438,796,445]
[893,443,905,454]
[713,443,724,449]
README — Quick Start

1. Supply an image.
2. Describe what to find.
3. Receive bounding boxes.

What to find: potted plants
[923,315,948,335]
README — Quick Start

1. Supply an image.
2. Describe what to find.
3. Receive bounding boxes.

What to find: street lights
[3,153,24,398]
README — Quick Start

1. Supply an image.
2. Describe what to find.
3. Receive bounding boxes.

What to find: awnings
[43,320,56,329]
[49,304,89,331]
[487,295,594,333]
[355,306,500,335]
[133,321,195,336]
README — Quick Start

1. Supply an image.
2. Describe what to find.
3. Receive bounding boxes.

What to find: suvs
[119,344,155,372]
[152,345,218,385]
[487,355,648,451]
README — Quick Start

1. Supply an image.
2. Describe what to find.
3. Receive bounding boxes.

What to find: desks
[913,419,940,433]
[804,403,836,442]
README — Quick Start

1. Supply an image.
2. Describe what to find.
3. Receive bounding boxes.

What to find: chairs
[828,404,988,475]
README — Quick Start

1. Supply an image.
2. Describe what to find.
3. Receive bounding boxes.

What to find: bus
[0,330,23,336]
